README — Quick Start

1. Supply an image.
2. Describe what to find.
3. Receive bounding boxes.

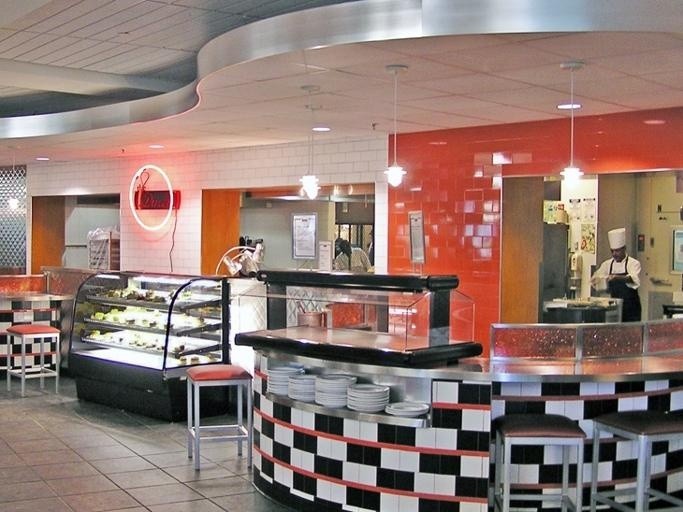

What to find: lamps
[384,66,407,186]
[313,106,331,132]
[560,62,585,183]
[300,85,318,201]
[7,146,19,210]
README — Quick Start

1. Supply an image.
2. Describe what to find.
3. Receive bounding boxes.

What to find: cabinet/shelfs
[68,269,232,420]
[239,271,483,366]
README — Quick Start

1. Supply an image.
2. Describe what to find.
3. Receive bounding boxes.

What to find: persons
[333,231,373,273]
[586,227,641,322]
[238,244,258,278]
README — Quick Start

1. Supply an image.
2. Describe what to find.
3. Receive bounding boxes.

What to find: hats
[608,227,626,250]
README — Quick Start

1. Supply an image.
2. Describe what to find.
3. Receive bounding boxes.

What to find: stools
[592,410,683,512]
[7,325,60,397]
[494,414,588,512]
[186,365,252,470]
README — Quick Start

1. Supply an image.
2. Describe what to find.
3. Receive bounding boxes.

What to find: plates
[267,367,429,419]
